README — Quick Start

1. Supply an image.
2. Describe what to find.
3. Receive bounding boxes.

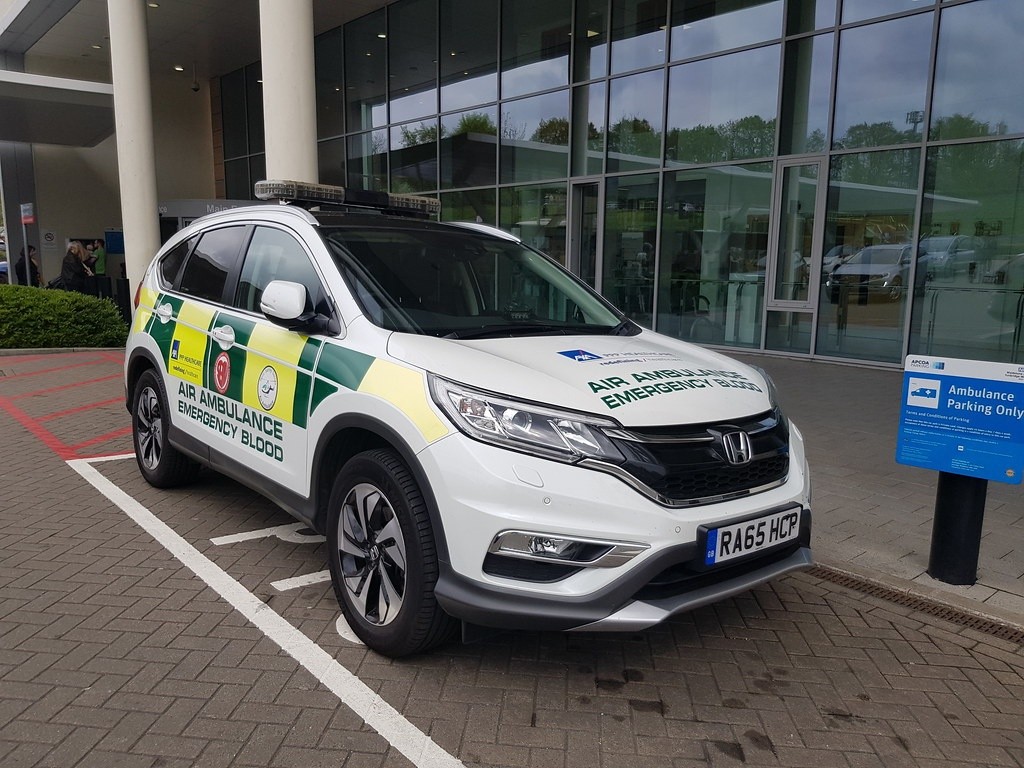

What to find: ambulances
[122,179,813,662]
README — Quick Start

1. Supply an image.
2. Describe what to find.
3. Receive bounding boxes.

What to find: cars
[734,234,1024,324]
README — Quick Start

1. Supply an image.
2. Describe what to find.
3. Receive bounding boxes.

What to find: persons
[61,239,106,296]
[15,245,45,288]
